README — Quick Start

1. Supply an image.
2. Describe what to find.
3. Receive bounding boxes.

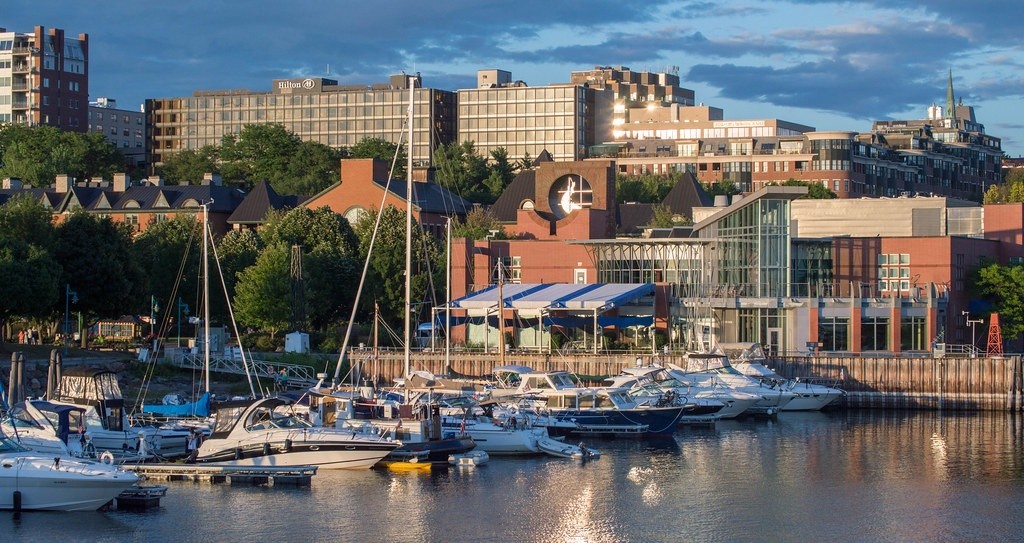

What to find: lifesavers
[507,404,520,417]
[100,451,115,467]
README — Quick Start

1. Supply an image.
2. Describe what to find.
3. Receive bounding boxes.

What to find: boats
[0,75,849,511]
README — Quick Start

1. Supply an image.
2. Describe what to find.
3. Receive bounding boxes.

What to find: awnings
[452,282,655,310]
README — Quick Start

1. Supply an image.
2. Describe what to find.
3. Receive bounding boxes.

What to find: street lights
[151,294,160,336]
[178,296,190,348]
[65,284,78,345]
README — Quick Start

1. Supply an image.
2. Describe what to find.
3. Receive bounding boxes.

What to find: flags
[459,417,466,435]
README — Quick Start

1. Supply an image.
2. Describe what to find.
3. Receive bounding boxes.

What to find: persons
[17,327,43,345]
[272,368,292,392]
[136,430,149,465]
[185,428,199,465]
